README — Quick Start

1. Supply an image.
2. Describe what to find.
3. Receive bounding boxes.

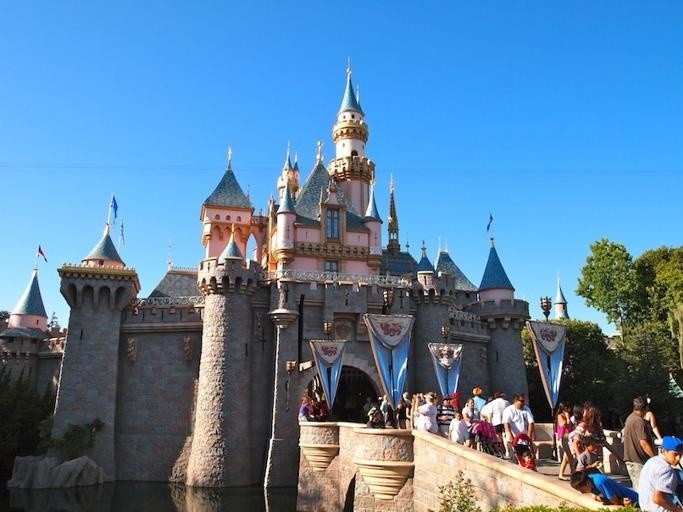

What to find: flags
[111,197,117,219]
[486,214,493,231]
[38,246,47,264]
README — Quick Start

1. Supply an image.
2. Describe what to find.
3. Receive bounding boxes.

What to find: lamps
[284,359,298,376]
[321,319,336,336]
[440,322,452,339]
[382,288,396,309]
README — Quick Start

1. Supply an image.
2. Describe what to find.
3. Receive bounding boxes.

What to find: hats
[473,388,482,395]
[442,394,453,401]
[655,436,683,452]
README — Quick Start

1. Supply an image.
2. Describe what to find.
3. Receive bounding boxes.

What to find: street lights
[324,320,334,416]
[383,288,396,420]
[441,324,449,396]
[540,296,554,422]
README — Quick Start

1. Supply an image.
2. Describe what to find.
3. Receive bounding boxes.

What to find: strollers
[510,434,537,472]
[472,422,504,459]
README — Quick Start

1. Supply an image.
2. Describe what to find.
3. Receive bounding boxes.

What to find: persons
[638,437,683,511]
[333,394,383,429]
[300,390,328,420]
[555,401,607,481]
[450,387,536,471]
[570,471,639,507]
[380,391,457,437]
[624,397,663,486]
